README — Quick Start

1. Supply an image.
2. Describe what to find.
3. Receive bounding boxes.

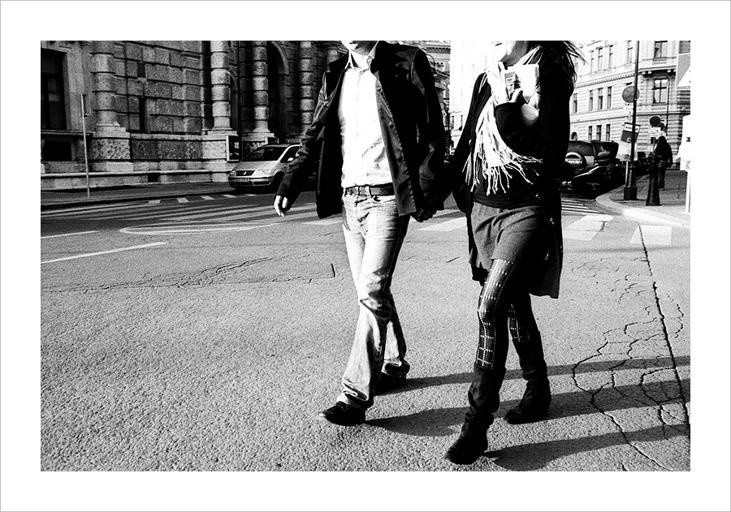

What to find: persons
[654,136,674,188]
[274,41,446,427]
[416,42,577,465]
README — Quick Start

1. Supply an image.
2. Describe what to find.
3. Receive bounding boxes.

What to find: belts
[341,182,395,199]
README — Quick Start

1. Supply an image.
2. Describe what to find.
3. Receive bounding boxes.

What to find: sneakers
[375,371,409,396]
[317,402,368,427]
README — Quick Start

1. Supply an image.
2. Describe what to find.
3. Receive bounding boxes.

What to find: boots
[505,331,553,424]
[441,362,507,466]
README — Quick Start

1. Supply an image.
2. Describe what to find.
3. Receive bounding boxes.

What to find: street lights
[651,73,670,128]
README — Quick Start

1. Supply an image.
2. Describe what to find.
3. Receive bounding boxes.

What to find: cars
[560,140,646,198]
[228,143,316,193]
